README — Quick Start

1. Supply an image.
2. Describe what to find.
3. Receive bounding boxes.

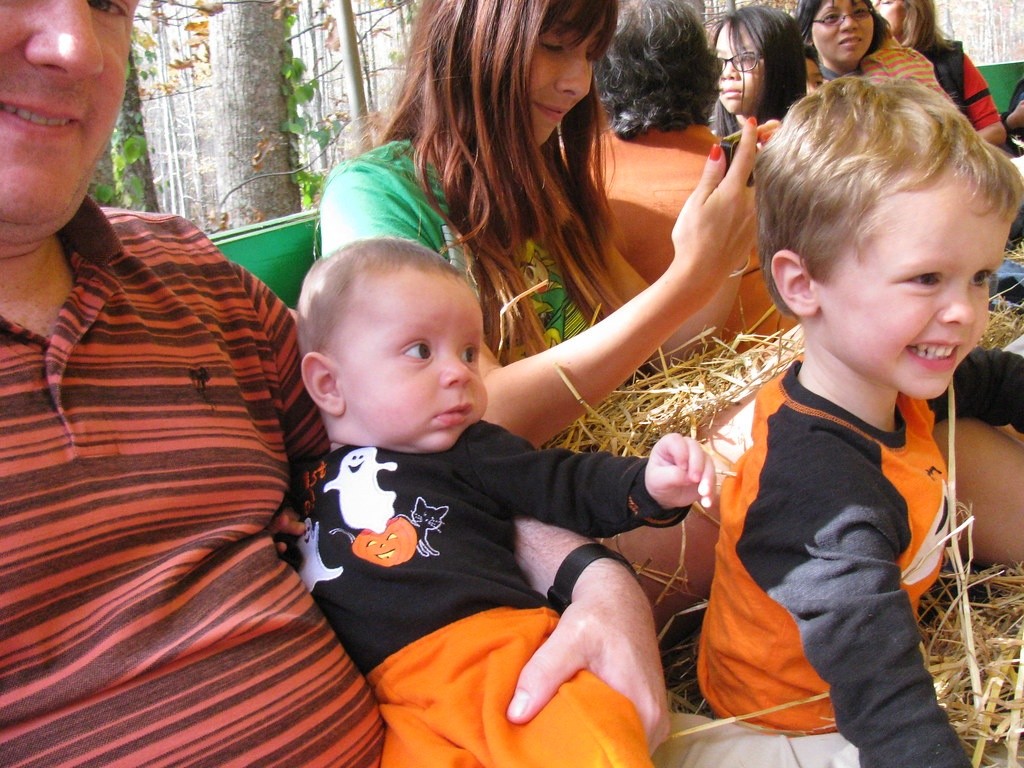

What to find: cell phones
[720,130,744,177]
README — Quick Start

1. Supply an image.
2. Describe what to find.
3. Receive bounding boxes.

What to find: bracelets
[545,542,637,618]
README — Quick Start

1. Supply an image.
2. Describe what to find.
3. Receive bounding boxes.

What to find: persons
[996,77,1024,157]
[802,42,824,99]
[0,0,861,768]
[697,72,1024,768]
[796,0,891,85]
[559,0,801,381]
[321,0,1024,644]
[264,236,718,768]
[870,0,1007,146]
[711,5,808,187]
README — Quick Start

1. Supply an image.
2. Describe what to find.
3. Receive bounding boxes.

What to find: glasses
[719,51,765,73]
[811,7,872,25]
